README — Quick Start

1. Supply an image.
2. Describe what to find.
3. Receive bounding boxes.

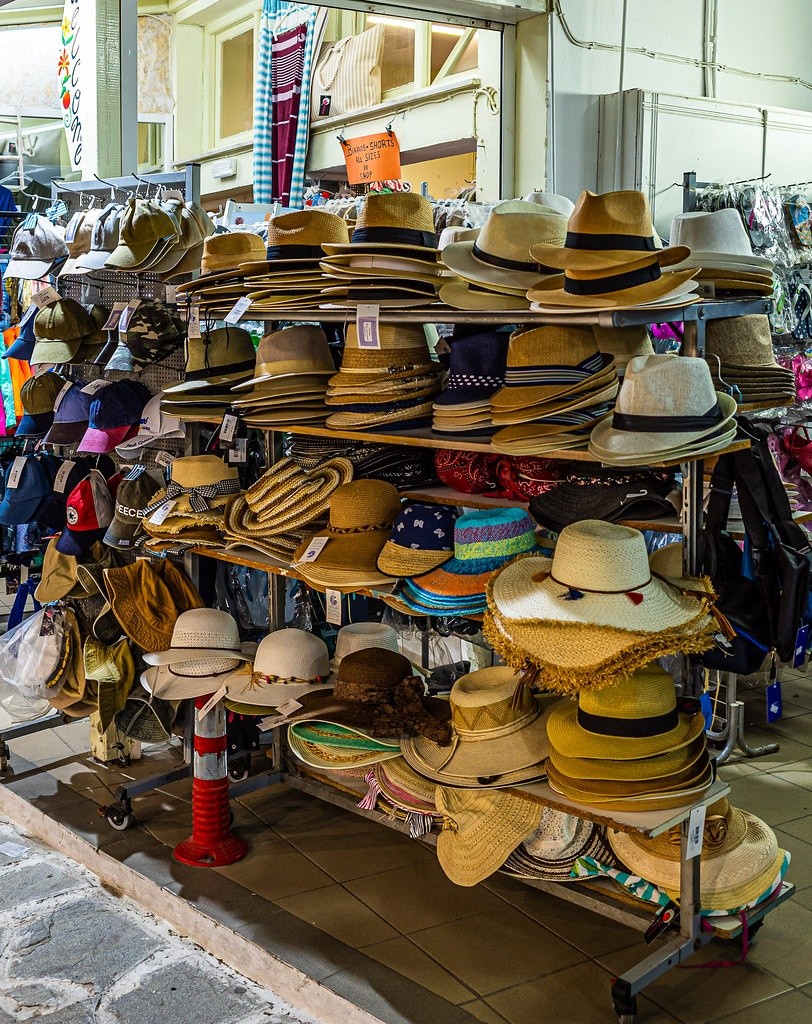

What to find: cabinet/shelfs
[0,172,811,1023]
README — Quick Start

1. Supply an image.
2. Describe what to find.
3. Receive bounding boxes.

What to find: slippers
[690,188,812,514]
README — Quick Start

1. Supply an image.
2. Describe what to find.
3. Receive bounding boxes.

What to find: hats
[0,191,805,917]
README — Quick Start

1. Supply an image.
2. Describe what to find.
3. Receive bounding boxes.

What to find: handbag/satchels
[310,23,385,123]
[0,129,61,186]
[691,416,812,676]
